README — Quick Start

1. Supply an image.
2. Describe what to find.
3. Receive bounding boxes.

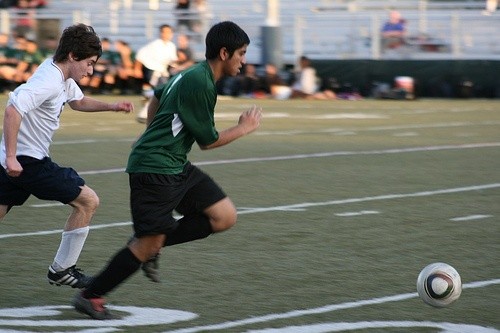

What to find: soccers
[416,261,462,308]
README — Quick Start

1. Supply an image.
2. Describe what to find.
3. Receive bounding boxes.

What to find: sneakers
[72,296,121,319]
[129,238,159,283]
[47,266,92,288]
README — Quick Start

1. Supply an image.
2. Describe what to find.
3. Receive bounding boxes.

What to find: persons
[0,24,135,289]
[1,33,342,101]
[134,24,179,124]
[75,21,264,320]
[378,8,407,53]
